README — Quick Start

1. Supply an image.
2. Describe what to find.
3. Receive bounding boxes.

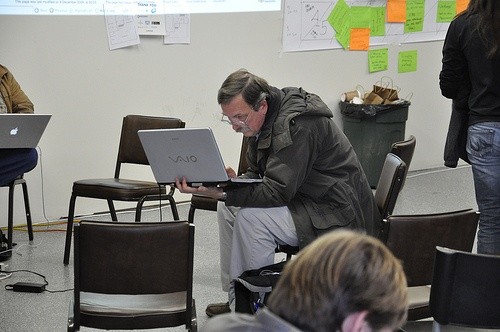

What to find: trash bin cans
[339,98,411,188]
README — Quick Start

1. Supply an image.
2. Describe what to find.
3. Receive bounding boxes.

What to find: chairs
[379,208,481,287]
[374,152,407,218]
[391,135,416,189]
[67,221,198,332]
[187,136,256,223]
[429,246,500,332]
[63,115,185,266]
[0,173,33,249]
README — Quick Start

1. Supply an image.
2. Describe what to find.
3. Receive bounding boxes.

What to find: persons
[438,0,500,255]
[175,69,382,316]
[201,228,408,332]
[0,64,38,259]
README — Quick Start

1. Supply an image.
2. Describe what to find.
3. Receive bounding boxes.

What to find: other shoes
[205,301,232,317]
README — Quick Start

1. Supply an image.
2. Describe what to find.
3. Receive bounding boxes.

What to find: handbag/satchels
[365,75,412,105]
[232,270,285,315]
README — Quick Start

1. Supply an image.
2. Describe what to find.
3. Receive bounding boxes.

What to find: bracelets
[222,188,227,200]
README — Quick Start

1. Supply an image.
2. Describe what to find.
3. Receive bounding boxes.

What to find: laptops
[138,128,263,187]
[0,114,52,148]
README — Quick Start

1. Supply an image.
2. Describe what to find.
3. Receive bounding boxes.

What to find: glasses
[221,100,264,126]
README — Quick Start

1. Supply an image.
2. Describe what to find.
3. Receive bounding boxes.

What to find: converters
[12,282,45,293]
